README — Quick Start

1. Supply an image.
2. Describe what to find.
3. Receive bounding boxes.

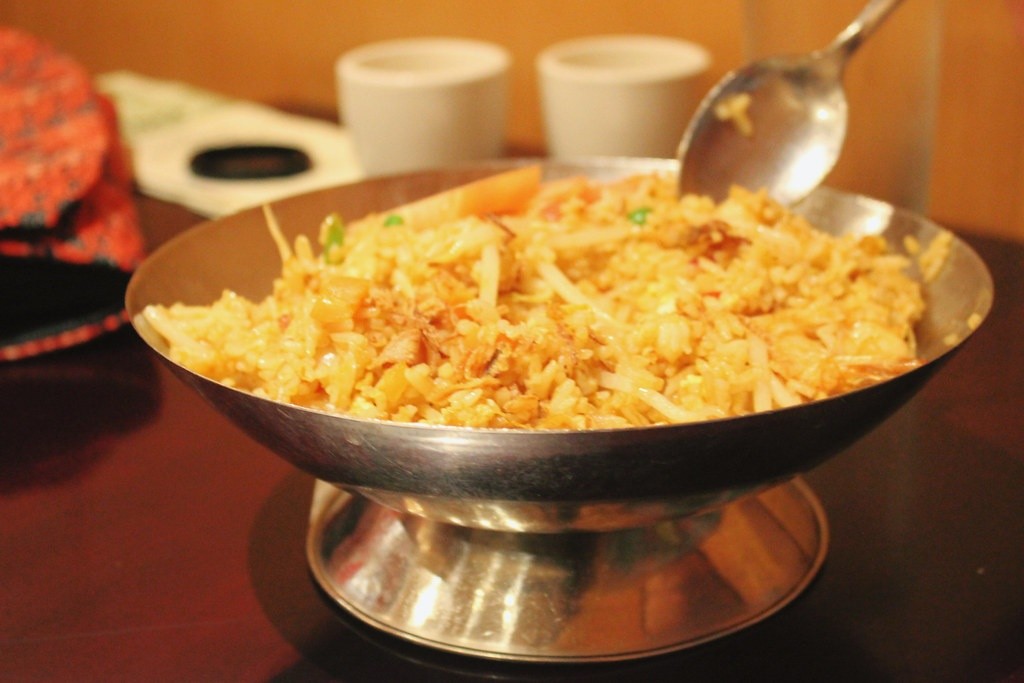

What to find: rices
[140,161,924,426]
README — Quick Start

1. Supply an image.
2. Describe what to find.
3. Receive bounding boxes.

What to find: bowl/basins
[126,152,992,662]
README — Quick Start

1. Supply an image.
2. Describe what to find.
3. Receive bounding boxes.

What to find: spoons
[676,1,904,213]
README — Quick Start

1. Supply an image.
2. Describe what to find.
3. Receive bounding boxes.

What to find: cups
[537,34,712,157]
[334,37,511,174]
